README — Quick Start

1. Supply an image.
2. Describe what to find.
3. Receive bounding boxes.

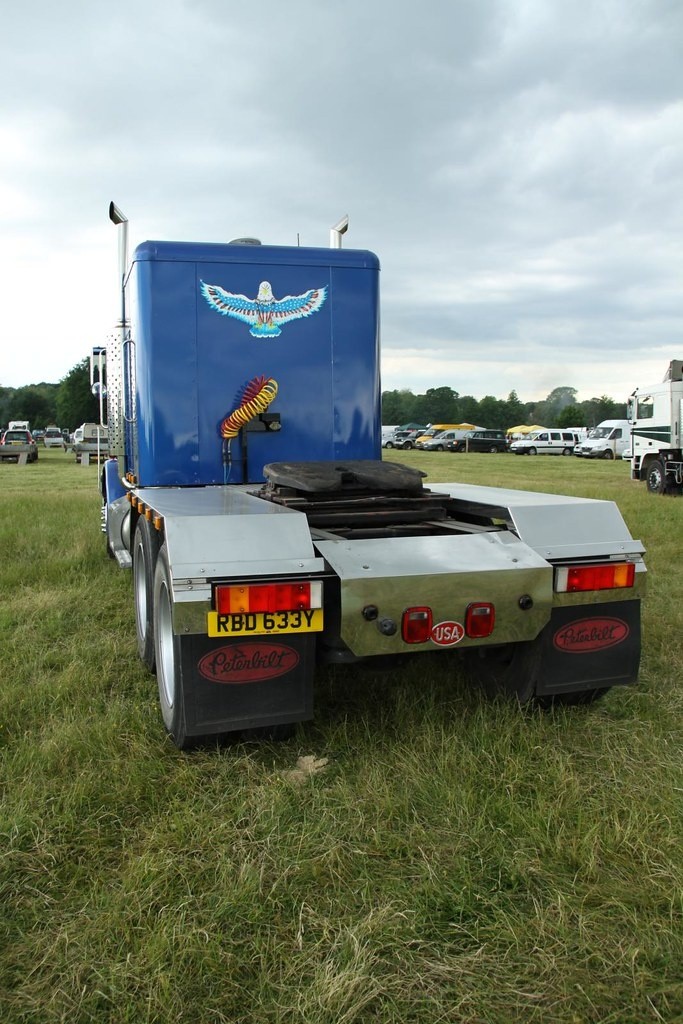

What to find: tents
[395,423,433,432]
[459,423,487,431]
[506,425,547,440]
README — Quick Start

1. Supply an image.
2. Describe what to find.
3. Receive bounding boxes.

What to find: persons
[61,428,70,452]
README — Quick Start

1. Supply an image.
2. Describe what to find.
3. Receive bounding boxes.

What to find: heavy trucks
[99,199,649,749]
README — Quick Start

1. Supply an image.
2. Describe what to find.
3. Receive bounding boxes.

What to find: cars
[0,423,108,462]
[380,359,683,494]
